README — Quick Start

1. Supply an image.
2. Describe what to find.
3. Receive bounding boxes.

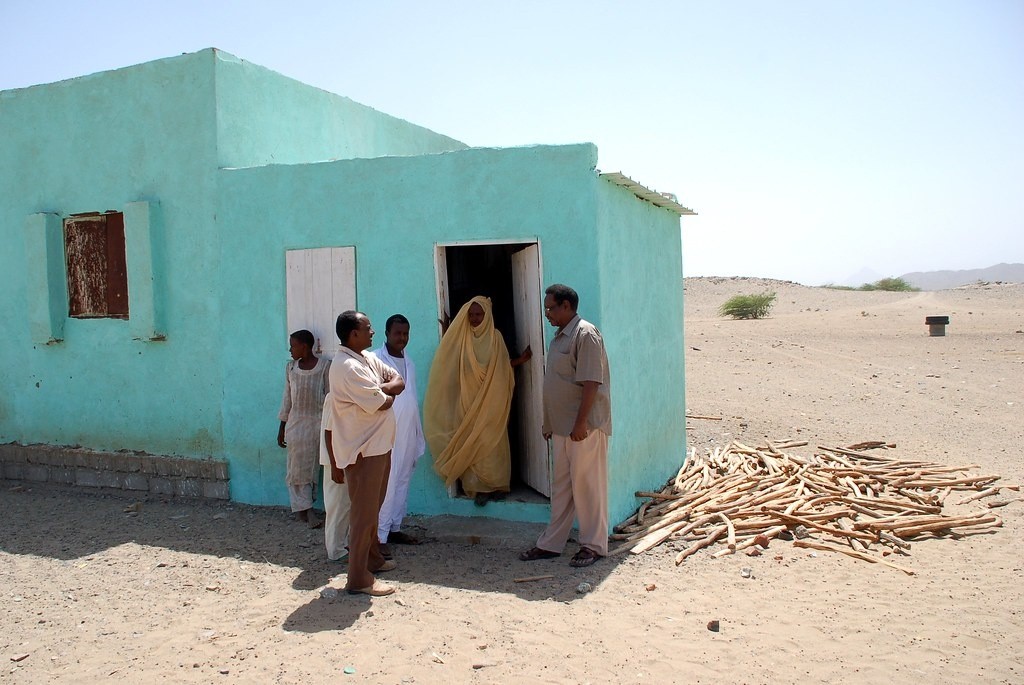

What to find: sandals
[519,548,562,560]
[568,547,601,567]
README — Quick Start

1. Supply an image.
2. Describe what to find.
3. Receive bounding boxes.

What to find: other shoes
[493,493,505,501]
[390,531,422,545]
[380,543,393,560]
[475,495,489,508]
[348,578,395,596]
[373,560,398,574]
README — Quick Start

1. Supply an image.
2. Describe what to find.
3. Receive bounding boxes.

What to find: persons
[438,296,532,506]
[278,310,420,596]
[519,286,611,567]
